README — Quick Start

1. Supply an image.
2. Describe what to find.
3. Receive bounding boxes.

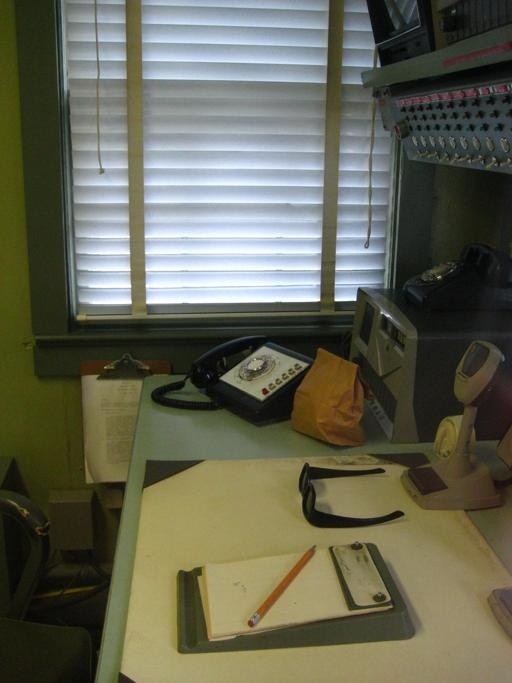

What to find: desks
[94,375,512,682]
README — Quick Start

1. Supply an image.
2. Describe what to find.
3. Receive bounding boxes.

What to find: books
[197,540,394,643]
[80,374,143,485]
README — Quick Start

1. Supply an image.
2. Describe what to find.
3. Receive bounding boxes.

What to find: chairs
[1,489,93,682]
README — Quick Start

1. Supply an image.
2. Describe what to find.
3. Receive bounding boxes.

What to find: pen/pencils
[247,544,319,628]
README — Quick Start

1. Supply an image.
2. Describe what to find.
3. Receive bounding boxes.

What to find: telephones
[189,334,314,427]
[402,242,512,311]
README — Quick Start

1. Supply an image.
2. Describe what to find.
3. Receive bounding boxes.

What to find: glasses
[300,462,404,527]
